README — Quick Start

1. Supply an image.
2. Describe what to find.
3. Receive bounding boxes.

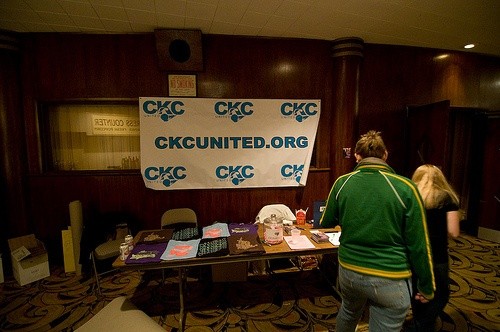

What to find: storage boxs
[7,233,51,286]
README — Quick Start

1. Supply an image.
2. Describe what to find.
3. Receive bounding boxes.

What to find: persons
[412,164,459,332]
[319,131,436,332]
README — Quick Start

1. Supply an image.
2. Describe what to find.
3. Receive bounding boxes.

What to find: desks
[112,217,343,332]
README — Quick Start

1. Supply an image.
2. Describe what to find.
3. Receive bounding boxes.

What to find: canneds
[120,242,129,261]
[125,235,133,251]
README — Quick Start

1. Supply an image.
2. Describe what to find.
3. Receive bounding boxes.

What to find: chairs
[255,203,301,276]
[88,211,143,299]
[160,207,198,230]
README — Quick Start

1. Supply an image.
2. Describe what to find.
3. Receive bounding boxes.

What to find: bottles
[263,214,284,245]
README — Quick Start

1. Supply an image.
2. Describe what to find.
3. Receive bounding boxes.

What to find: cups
[291,229,301,244]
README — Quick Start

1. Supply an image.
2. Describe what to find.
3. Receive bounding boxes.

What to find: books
[312,233,329,243]
